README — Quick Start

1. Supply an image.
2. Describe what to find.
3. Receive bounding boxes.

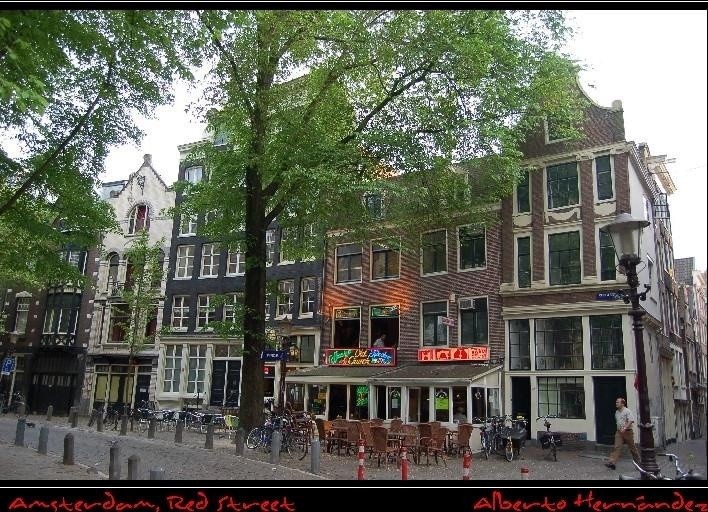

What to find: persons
[604,398,640,471]
[452,407,467,425]
[372,334,385,348]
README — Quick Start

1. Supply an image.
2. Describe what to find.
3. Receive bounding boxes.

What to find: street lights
[267,330,300,414]
[598,210,659,480]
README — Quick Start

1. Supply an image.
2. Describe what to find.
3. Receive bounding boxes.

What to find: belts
[617,429,632,432]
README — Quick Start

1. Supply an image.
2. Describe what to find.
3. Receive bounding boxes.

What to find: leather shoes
[605,462,616,471]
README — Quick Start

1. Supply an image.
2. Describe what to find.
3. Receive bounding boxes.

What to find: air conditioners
[458,299,475,311]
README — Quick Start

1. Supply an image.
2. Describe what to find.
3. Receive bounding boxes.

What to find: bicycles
[87,400,120,430]
[472,414,528,462]
[536,413,560,462]
[616,452,703,482]
[0,391,30,418]
[246,414,308,460]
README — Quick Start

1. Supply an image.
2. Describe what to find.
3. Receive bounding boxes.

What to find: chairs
[318,414,475,466]
